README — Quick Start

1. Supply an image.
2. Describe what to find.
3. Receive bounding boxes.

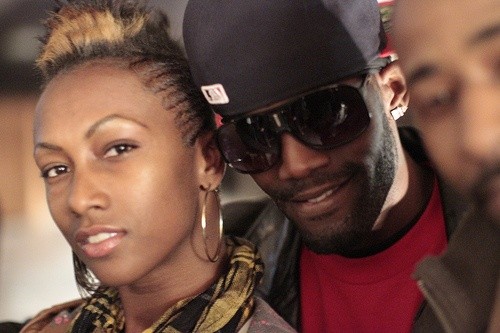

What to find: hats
[183,0,386,122]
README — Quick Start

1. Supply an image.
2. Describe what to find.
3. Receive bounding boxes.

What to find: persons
[19,0,298,333]
[391,0,500,333]
[183,0,464,333]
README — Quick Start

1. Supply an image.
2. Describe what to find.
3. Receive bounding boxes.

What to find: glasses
[214,72,379,174]
